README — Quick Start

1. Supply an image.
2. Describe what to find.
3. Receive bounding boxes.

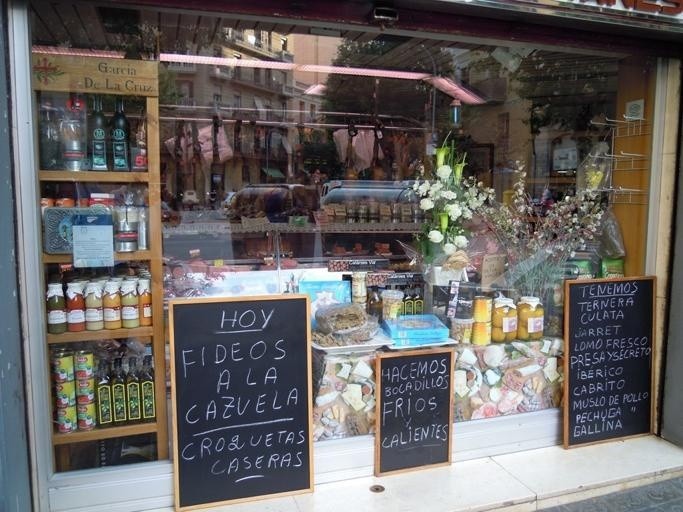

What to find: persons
[156,137,434,274]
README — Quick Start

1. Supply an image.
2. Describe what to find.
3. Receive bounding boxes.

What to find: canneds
[135,206,149,250]
[451,296,544,345]
[49,349,96,433]
[40,198,90,217]
[115,206,138,252]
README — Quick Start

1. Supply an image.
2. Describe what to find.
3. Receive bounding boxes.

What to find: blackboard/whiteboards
[375,347,455,477]
[168,294,314,511]
[564,276,657,450]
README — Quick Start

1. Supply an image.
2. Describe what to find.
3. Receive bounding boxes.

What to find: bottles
[40,94,148,171]
[381,289,403,321]
[47,269,152,334]
[95,355,156,428]
[346,200,433,224]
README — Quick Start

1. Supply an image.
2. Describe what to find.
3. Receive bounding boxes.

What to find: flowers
[414,144,604,298]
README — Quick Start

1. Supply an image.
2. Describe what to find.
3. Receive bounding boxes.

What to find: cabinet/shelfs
[167,198,425,268]
[35,59,167,466]
